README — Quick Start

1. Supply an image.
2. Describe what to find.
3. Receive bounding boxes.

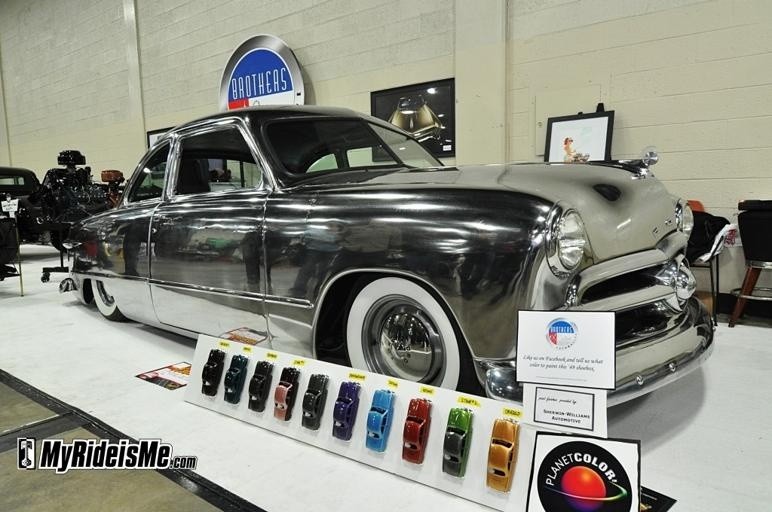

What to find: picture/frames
[146,126,177,179]
[370,77,455,162]
[544,110,615,162]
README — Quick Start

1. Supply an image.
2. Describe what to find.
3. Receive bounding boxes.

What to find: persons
[563,137,576,163]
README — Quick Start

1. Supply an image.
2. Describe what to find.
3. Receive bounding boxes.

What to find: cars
[66,102,716,412]
[200,347,522,495]
[0,149,128,283]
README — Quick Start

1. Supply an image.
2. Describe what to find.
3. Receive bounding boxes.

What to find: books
[484,416,521,495]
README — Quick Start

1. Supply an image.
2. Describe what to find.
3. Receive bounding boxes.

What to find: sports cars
[387,92,445,145]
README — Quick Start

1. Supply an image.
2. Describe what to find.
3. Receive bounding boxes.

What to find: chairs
[684,199,772,328]
